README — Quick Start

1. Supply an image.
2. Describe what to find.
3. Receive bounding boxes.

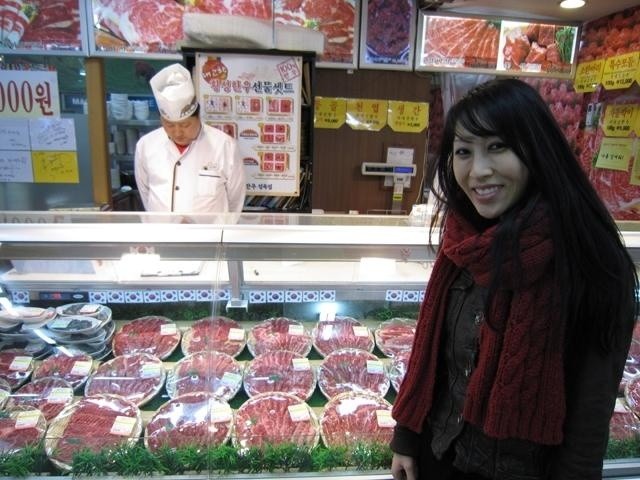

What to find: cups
[113,128,139,155]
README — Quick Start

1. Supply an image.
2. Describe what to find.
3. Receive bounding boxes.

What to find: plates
[311,316,375,356]
[46,395,141,470]
[317,347,390,401]
[112,315,181,362]
[166,351,242,402]
[232,390,319,453]
[246,316,312,360]
[2,351,34,388]
[4,406,46,458]
[34,350,95,393]
[242,350,317,404]
[320,392,394,450]
[181,317,247,361]
[1,377,12,409]
[3,303,115,360]
[389,357,410,391]
[375,318,420,359]
[86,352,166,406]
[146,393,233,450]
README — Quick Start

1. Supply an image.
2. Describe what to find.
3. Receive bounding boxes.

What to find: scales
[362,162,417,215]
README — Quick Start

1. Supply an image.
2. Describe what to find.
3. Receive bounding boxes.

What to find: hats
[149,62,198,123]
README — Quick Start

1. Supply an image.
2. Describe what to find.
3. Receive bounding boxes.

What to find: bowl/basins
[103,92,149,123]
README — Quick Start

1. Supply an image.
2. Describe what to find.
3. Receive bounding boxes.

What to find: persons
[391,77,640,480]
[133,63,248,224]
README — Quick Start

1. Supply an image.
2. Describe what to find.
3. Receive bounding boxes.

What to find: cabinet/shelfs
[106,92,158,190]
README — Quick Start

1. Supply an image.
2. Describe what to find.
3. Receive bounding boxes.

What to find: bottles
[108,141,121,191]
